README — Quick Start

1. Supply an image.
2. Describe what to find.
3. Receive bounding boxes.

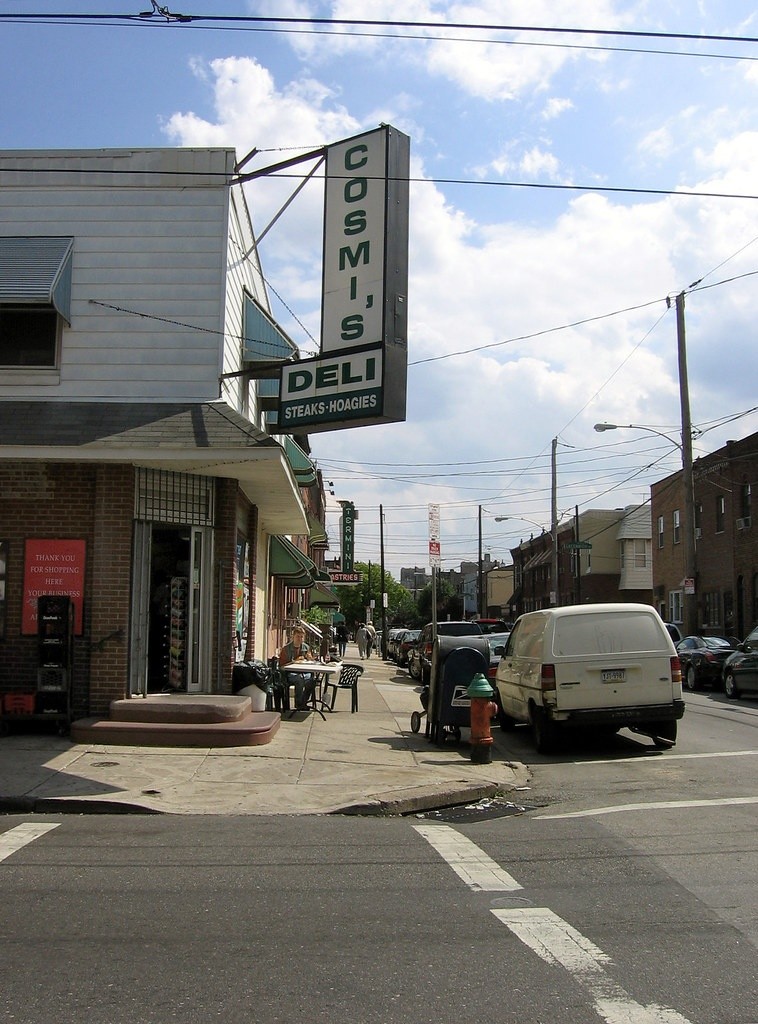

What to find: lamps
[325,489,335,495]
[323,480,334,486]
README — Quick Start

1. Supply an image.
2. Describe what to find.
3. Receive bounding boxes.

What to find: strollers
[410,647,490,745]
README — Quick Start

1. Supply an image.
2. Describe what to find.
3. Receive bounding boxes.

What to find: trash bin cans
[234,665,273,713]
[432,647,489,751]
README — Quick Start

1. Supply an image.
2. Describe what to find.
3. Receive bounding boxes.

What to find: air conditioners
[736,516,752,530]
[290,602,299,618]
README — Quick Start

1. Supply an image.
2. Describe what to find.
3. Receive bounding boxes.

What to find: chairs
[321,664,364,713]
[244,658,286,711]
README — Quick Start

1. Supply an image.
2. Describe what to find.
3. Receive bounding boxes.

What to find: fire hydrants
[467,673,498,764]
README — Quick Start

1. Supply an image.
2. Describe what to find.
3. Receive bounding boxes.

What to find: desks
[283,662,343,721]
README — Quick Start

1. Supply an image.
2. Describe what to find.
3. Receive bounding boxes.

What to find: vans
[495,602,685,755]
[664,623,683,649]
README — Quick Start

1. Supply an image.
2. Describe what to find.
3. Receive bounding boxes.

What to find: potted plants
[318,610,336,633]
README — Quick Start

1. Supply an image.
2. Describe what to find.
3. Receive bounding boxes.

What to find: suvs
[409,621,488,680]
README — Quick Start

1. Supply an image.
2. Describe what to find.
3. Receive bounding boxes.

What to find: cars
[372,630,383,648]
[469,618,512,636]
[483,632,512,685]
[722,625,758,699]
[674,635,743,693]
[387,628,423,667]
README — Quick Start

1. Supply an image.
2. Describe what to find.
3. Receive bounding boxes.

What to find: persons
[355,621,376,659]
[279,626,316,709]
[336,621,349,657]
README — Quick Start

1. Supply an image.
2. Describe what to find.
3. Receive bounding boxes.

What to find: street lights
[495,516,559,607]
[594,423,701,636]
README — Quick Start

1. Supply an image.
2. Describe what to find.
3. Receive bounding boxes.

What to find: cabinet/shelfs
[36,594,75,713]
[169,575,188,688]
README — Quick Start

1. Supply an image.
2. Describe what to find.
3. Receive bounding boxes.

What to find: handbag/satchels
[366,629,372,639]
[372,644,376,649]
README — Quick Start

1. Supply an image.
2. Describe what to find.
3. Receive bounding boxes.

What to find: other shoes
[295,703,309,710]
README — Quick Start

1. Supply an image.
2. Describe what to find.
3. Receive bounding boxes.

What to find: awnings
[310,588,340,608]
[243,289,299,361]
[295,473,317,487]
[332,610,345,622]
[285,435,316,475]
[0,237,73,325]
[308,514,327,546]
[506,588,522,604]
[255,379,281,397]
[266,410,278,424]
[271,535,330,589]
